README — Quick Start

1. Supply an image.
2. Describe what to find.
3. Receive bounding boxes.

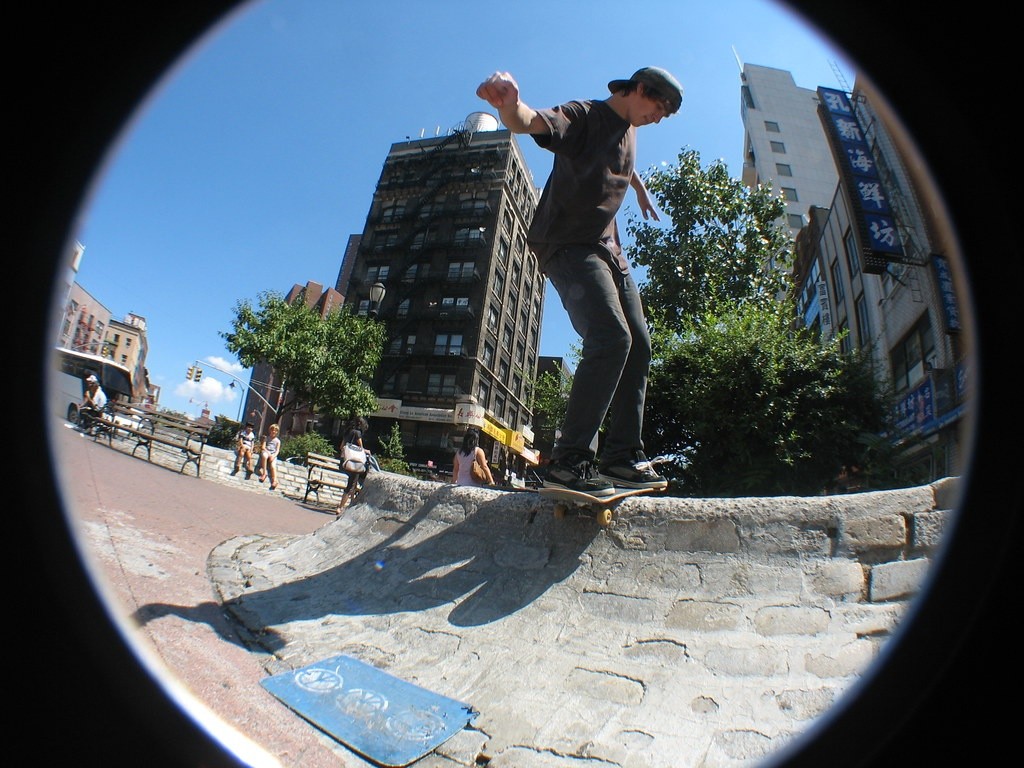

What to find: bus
[45,347,133,428]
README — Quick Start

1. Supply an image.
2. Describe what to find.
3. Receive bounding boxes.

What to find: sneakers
[542,459,615,498]
[598,450,670,489]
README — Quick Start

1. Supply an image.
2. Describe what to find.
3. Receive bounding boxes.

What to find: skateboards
[538,480,667,527]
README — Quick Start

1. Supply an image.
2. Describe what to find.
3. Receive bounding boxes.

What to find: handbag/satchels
[470,448,489,484]
[343,444,368,475]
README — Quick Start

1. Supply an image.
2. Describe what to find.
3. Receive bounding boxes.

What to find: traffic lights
[185,366,194,381]
[106,346,110,359]
[102,345,106,358]
[195,369,203,382]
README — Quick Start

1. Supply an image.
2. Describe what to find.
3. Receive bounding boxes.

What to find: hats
[86,374,98,383]
[609,69,684,110]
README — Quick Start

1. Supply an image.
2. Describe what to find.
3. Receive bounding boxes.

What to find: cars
[100,406,149,434]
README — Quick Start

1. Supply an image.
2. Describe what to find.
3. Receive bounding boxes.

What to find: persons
[334,416,372,514]
[849,465,863,491]
[259,424,280,489]
[73,375,107,433]
[476,66,683,495]
[451,431,495,489]
[915,395,925,422]
[234,420,254,473]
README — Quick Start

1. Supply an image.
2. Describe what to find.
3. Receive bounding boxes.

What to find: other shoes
[235,465,241,472]
[259,474,267,482]
[271,480,279,488]
[248,467,254,474]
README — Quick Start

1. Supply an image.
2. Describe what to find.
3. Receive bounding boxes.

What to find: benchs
[93,407,212,477]
[303,451,363,508]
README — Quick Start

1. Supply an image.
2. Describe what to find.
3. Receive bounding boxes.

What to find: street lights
[229,379,245,423]
